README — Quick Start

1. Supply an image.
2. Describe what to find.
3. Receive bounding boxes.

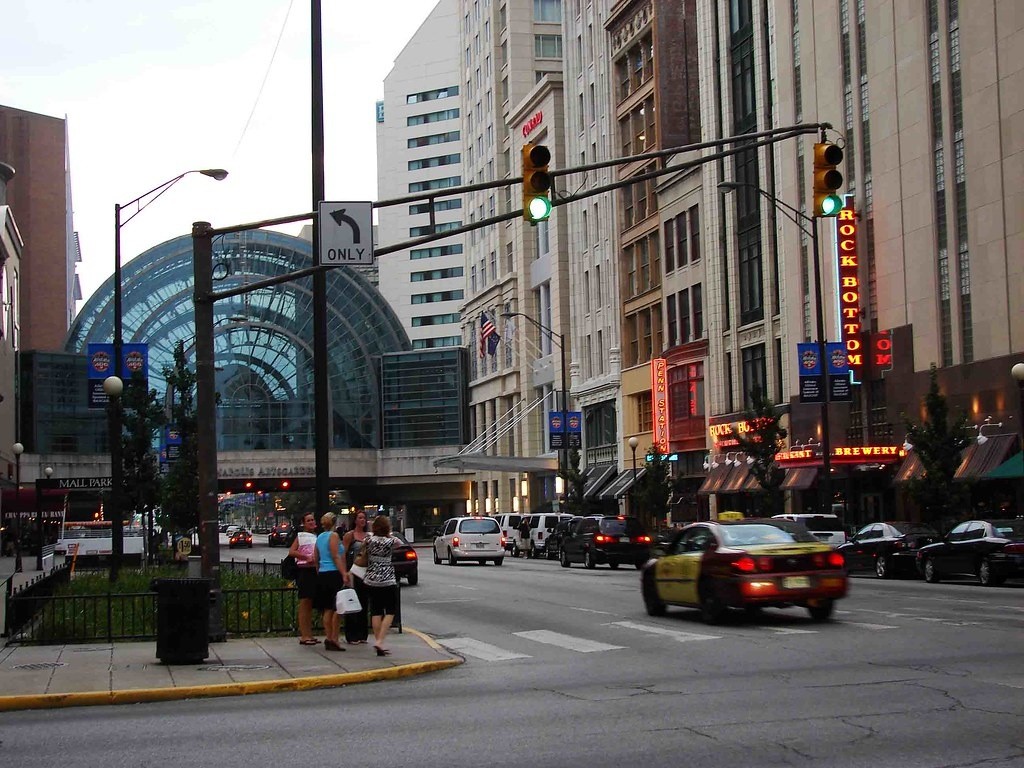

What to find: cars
[228,531,253,549]
[366,531,418,585]
[638,511,851,625]
[267,527,288,547]
[914,517,1024,587]
[837,520,945,580]
[217,523,274,537]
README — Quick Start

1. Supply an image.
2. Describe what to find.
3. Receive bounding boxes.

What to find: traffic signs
[317,199,374,265]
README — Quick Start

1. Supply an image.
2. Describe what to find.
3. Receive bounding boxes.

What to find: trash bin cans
[150,577,214,663]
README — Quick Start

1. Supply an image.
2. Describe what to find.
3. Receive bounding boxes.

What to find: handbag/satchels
[336,583,362,614]
[351,563,369,579]
[281,554,298,581]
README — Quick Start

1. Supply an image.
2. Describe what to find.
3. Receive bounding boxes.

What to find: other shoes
[350,639,360,644]
[324,640,347,651]
[374,646,392,656]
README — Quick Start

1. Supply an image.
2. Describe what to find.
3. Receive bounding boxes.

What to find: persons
[288,512,321,645]
[517,518,531,560]
[3,527,16,557]
[360,515,401,656]
[343,509,371,645]
[313,512,351,651]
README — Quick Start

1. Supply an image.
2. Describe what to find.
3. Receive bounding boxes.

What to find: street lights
[716,180,833,514]
[499,311,569,514]
[102,375,124,584]
[11,442,24,573]
[628,437,640,516]
[113,168,229,379]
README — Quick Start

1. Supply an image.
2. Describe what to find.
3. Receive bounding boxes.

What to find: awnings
[560,465,617,500]
[695,457,776,494]
[889,430,1017,485]
[593,468,648,500]
[779,467,819,491]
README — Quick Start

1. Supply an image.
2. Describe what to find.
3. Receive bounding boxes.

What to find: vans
[488,512,653,571]
[432,513,506,566]
[770,513,848,548]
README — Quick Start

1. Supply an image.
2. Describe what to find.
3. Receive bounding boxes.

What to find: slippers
[299,638,322,645]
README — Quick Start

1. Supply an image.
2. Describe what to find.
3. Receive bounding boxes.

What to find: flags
[479,311,501,360]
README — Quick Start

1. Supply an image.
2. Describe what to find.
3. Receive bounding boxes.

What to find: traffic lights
[523,143,554,227]
[813,141,844,219]
